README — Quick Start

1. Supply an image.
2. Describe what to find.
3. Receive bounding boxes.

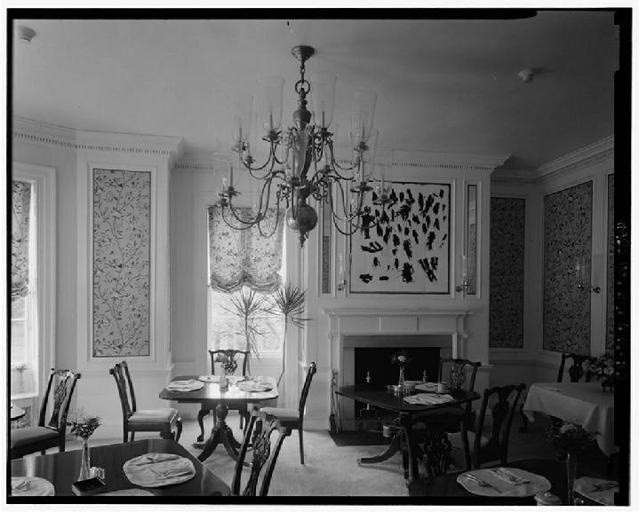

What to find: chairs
[207,350,251,434]
[331,349,630,504]
[231,408,291,502]
[107,359,184,444]
[8,367,81,466]
[259,359,320,464]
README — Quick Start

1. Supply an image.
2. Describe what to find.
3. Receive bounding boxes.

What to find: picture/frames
[348,179,451,294]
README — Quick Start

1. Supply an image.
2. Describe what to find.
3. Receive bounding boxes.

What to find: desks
[161,374,279,466]
[9,438,231,502]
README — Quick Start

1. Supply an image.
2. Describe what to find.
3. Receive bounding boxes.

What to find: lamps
[217,46,393,249]
